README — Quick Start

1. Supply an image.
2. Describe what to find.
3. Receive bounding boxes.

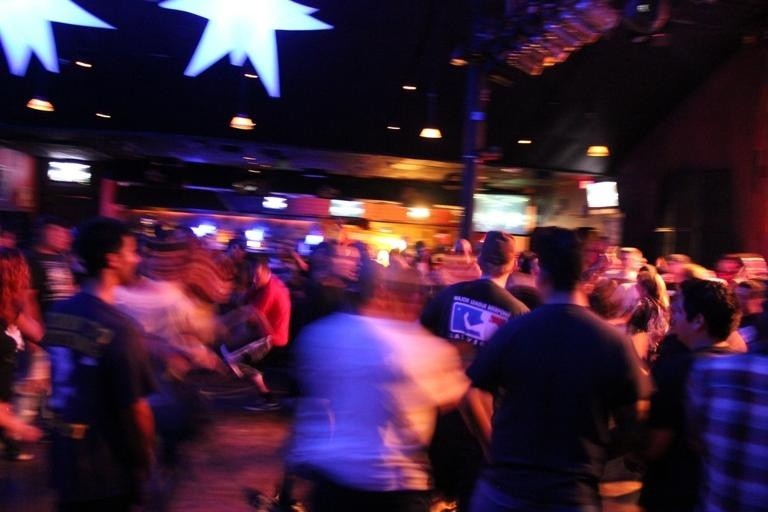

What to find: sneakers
[243,399,281,414]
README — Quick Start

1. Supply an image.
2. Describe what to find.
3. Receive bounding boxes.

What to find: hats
[482,230,516,264]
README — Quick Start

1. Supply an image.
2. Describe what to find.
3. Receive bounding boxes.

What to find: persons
[0,178,767,512]
